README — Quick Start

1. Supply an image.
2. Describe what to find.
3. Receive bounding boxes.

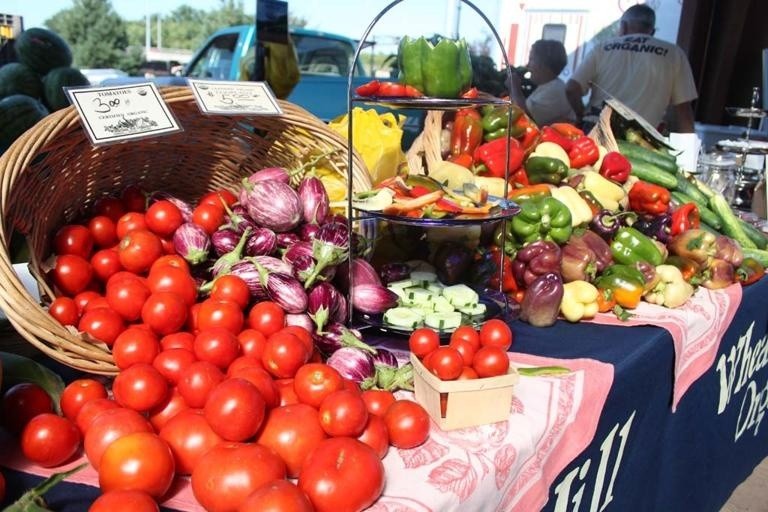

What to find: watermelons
[0,28,91,156]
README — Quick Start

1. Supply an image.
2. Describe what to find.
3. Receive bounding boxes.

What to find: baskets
[0,81,379,380]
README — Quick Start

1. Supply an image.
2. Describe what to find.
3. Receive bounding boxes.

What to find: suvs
[135,58,185,78]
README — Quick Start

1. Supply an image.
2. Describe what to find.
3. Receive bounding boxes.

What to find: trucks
[186,21,403,126]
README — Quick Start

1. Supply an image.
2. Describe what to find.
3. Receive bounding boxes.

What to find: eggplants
[145,167,415,394]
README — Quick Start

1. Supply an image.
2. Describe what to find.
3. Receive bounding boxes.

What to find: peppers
[356,35,764,328]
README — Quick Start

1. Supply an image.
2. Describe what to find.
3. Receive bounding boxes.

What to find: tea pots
[698,155,737,211]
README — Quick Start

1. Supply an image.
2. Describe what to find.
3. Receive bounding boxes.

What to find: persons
[502,38,576,129]
[563,3,699,136]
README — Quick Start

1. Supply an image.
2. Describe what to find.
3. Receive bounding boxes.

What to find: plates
[356,90,503,113]
[356,297,502,338]
[713,144,767,155]
[722,106,767,118]
[352,192,523,228]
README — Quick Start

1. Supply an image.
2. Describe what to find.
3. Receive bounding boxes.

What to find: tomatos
[409,320,512,418]
[1,186,430,512]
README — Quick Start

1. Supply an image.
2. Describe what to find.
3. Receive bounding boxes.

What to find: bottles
[738,166,767,218]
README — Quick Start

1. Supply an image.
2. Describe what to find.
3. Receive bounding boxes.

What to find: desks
[0,244,768,512]
